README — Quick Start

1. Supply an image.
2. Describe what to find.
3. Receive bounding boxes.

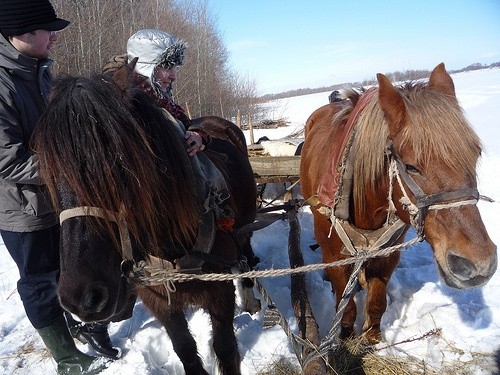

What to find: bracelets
[198,130,209,144]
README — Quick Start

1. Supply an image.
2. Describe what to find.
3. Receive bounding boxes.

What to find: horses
[28,56,262,375]
[300,61,498,352]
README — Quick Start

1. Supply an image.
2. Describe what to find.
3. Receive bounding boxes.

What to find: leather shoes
[76,320,122,360]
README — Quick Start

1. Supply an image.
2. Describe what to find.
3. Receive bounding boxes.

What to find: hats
[0,0,71,37]
[127,29,186,99]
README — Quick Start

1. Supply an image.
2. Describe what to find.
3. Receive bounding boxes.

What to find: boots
[64,311,89,344]
[35,314,112,375]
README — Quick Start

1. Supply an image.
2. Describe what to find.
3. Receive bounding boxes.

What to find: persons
[75,30,210,360]
[0,0,112,375]
